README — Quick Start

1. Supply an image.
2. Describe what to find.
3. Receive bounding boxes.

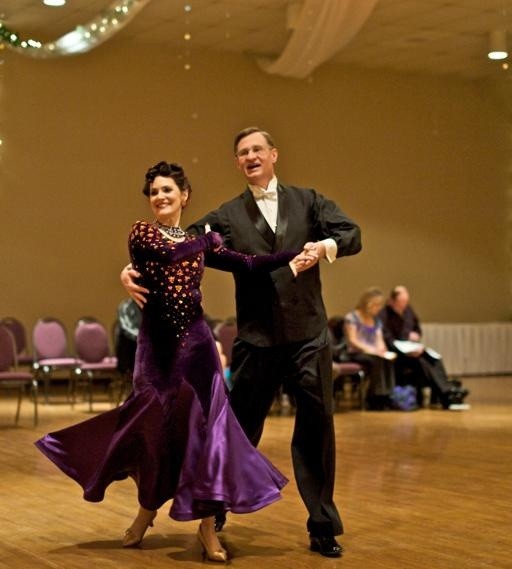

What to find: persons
[343,285,398,411]
[31,161,318,564]
[120,126,363,557]
[384,285,468,410]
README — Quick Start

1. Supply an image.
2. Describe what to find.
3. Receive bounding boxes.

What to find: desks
[420,321,512,377]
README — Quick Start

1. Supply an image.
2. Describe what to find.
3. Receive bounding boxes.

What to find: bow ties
[252,184,278,202]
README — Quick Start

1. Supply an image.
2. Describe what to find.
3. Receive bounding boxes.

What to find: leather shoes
[309,537,344,556]
[215,509,229,532]
[441,386,468,408]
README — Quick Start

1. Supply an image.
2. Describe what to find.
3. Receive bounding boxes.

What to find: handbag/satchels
[332,342,350,363]
[388,385,419,410]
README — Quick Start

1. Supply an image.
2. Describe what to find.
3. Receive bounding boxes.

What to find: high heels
[196,522,228,562]
[123,509,157,547]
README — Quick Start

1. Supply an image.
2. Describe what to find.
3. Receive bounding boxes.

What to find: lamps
[487,30,510,61]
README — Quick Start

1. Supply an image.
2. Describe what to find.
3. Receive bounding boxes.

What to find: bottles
[421,388,431,409]
[279,394,291,417]
[343,377,351,401]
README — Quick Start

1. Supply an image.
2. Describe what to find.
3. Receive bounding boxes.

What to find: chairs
[74,317,122,411]
[31,317,81,403]
[326,318,367,412]
[0,325,40,429]
[1,317,38,400]
[215,319,238,370]
